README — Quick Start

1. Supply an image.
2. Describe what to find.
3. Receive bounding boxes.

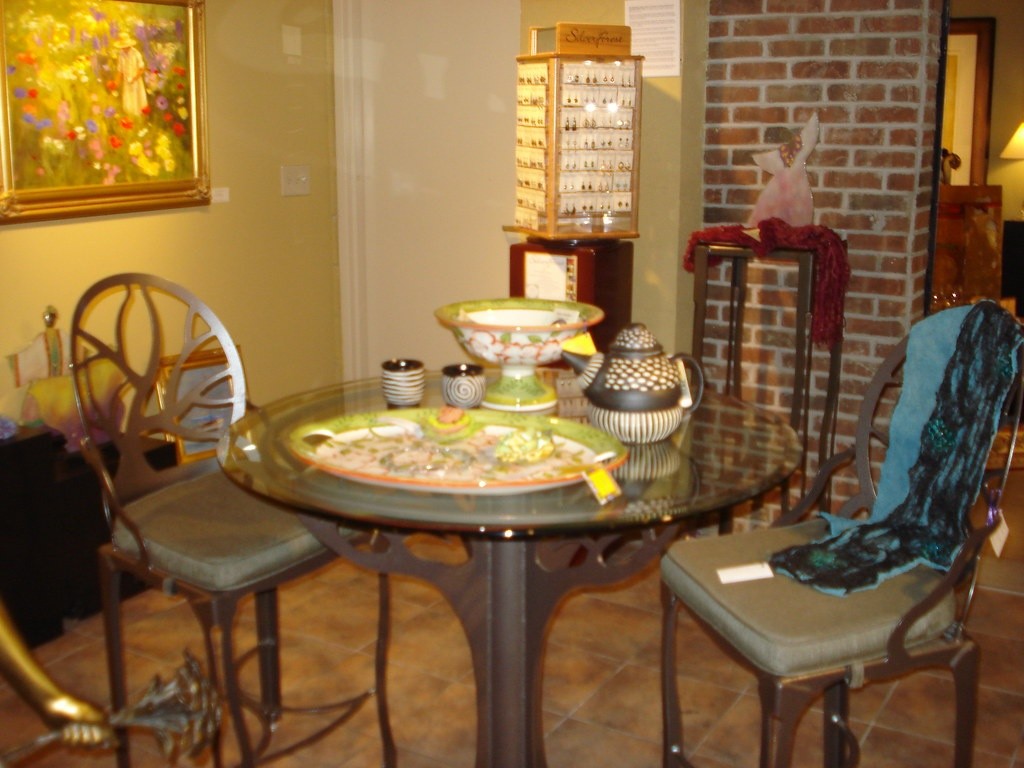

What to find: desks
[213,366,805,768]
[692,240,846,536]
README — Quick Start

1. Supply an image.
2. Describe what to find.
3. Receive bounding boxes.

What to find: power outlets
[280,165,310,197]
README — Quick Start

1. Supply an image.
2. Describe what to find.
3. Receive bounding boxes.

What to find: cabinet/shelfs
[0,418,178,648]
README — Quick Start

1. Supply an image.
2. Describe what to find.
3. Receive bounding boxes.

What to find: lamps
[999,123,1024,215]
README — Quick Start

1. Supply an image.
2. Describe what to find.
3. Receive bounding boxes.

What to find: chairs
[661,307,1024,768]
[69,274,400,768]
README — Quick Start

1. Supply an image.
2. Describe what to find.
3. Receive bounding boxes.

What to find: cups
[379,358,426,408]
[441,363,485,408]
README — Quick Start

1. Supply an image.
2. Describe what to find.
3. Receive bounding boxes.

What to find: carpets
[987,427,1024,470]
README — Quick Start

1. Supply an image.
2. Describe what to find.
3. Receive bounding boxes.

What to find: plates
[286,407,629,498]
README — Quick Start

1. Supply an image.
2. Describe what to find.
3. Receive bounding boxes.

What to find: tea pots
[560,322,705,447]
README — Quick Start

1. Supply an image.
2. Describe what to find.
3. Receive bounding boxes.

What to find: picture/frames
[153,344,251,466]
[0,0,212,227]
